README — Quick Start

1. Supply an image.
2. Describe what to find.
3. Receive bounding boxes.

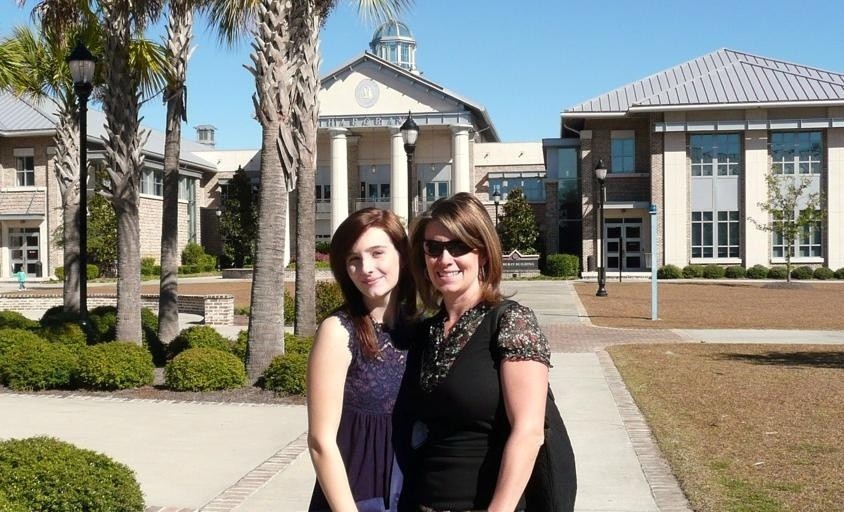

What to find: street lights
[398,109,420,235]
[592,156,609,299]
[491,188,502,230]
[62,38,100,333]
[215,206,222,253]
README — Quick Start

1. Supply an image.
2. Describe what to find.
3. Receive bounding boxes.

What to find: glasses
[422,238,472,257]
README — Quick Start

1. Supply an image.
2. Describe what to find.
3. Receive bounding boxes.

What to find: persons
[390,192,553,511]
[304,206,428,512]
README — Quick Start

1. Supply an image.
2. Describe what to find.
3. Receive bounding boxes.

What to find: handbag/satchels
[528,385,577,512]
